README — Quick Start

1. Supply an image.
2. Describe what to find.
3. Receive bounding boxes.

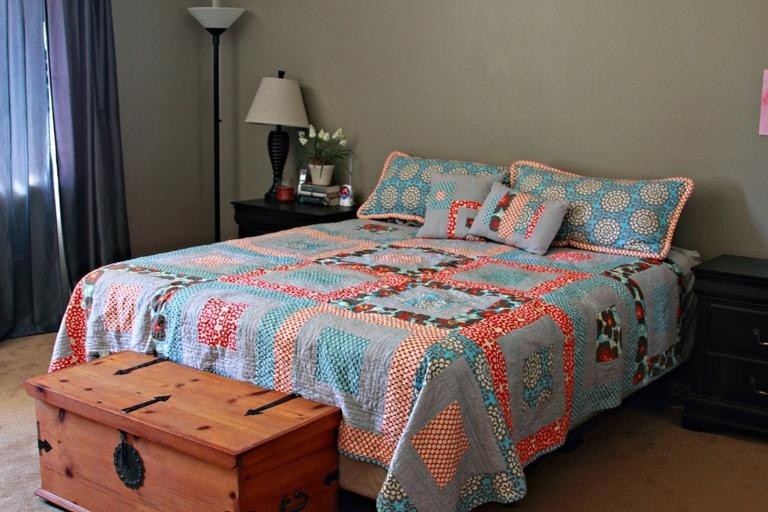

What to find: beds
[48,217,703,512]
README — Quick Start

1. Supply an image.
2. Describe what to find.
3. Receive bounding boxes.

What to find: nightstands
[680,254,768,441]
[230,197,356,239]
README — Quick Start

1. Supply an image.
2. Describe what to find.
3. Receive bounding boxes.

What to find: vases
[308,164,335,186]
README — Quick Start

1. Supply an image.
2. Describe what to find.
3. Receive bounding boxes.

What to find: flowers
[295,123,352,178]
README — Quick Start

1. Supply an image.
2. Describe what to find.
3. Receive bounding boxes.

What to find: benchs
[23,351,342,512]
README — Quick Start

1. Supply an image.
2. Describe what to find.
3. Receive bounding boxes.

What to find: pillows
[416,172,503,242]
[509,159,695,260]
[468,182,571,255]
[356,151,510,226]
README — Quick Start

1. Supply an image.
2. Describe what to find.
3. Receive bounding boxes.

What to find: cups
[340,185,353,206]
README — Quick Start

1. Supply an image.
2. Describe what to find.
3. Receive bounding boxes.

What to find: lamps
[186,0,247,243]
[244,70,310,202]
